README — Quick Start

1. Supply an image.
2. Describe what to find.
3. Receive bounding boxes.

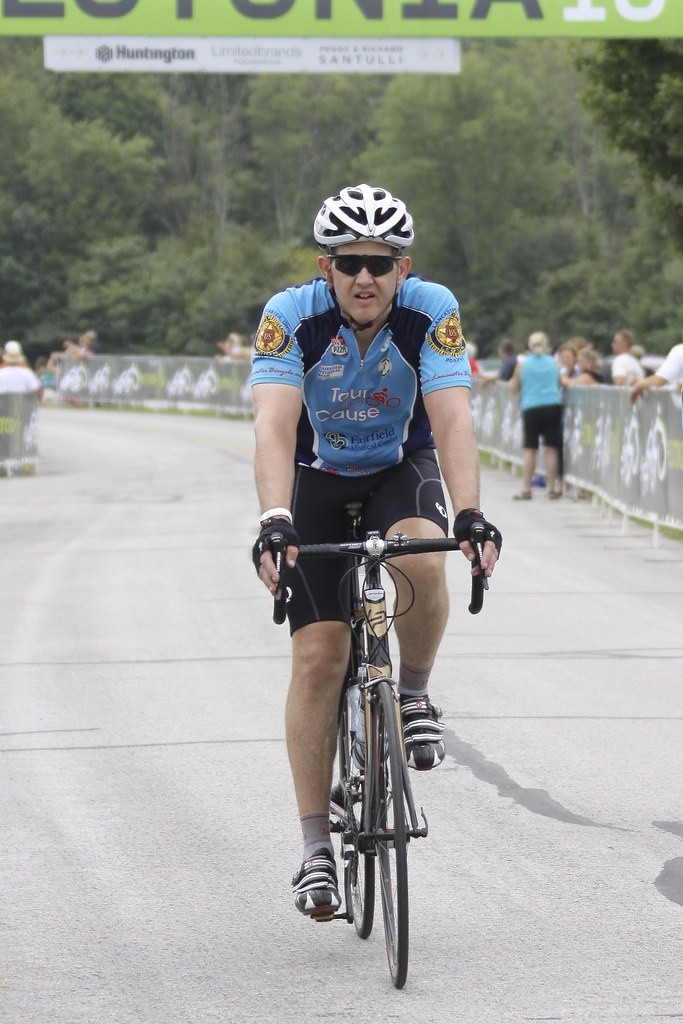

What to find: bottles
[349,695,373,771]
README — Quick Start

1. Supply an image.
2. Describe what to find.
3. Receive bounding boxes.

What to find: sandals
[512,491,531,499]
[548,491,561,499]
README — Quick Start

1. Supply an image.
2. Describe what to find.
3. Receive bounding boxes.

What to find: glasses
[327,254,403,277]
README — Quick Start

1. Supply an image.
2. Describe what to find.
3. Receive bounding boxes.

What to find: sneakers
[291,847,342,915]
[400,693,445,771]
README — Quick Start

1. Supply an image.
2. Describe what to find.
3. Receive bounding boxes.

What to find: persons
[629,342,683,404]
[465,329,646,391]
[215,332,254,365]
[496,330,571,501]
[0,329,100,409]
[248,182,502,915]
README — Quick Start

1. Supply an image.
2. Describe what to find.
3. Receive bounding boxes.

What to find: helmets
[313,184,415,254]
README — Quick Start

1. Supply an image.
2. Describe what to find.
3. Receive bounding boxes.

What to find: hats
[2,340,25,361]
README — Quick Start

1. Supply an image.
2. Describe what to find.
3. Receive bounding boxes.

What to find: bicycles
[268,520,491,990]
